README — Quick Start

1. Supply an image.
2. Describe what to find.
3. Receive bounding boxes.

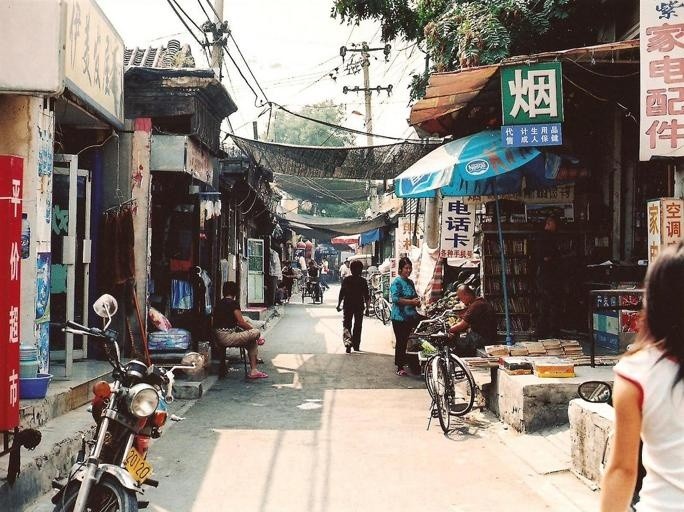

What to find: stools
[219,345,247,379]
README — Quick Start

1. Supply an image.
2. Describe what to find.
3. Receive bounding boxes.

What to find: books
[485,238,533,331]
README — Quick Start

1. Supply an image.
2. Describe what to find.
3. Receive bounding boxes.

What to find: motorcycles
[50,294,204,512]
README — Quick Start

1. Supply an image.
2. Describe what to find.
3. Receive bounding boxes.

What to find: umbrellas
[392,125,615,345]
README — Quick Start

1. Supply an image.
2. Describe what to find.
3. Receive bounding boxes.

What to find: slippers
[395,369,407,377]
[247,371,269,379]
[256,337,265,346]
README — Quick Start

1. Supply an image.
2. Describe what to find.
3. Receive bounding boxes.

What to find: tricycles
[302,276,325,304]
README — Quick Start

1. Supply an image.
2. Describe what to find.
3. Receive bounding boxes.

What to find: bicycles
[414,310,479,431]
[363,279,391,325]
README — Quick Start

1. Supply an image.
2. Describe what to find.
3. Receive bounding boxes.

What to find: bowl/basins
[19,372,54,399]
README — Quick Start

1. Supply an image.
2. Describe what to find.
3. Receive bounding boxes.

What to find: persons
[390,257,422,377]
[298,252,330,302]
[448,284,499,356]
[282,260,297,302]
[337,259,371,353]
[599,235,684,512]
[529,214,585,340]
[213,280,270,380]
[269,247,283,306]
